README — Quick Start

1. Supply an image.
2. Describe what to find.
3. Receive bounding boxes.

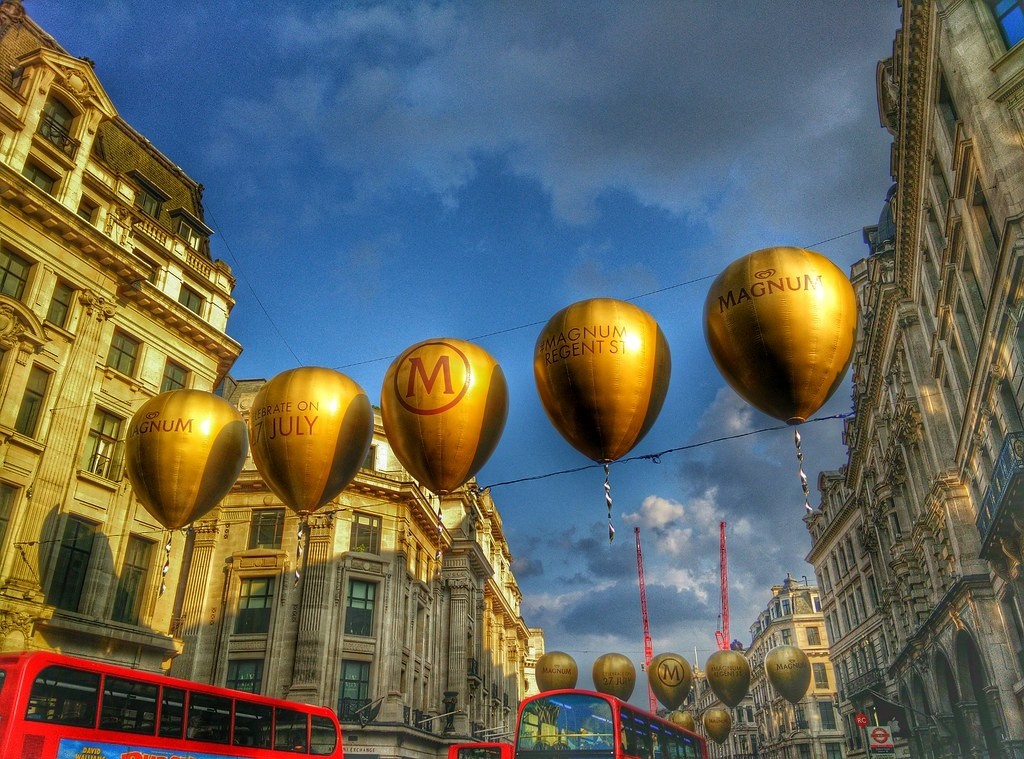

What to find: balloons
[125,389,249,530]
[667,711,695,732]
[380,338,509,495]
[764,645,811,705]
[648,652,692,710]
[535,651,578,693]
[704,708,731,745]
[705,650,750,709]
[248,366,374,515]
[702,246,860,425]
[533,297,672,463]
[592,653,636,702]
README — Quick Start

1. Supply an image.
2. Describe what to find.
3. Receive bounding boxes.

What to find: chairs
[167,727,182,736]
[60,712,79,723]
[532,740,550,751]
[120,724,134,730]
[139,722,153,732]
[552,740,570,750]
[44,714,59,721]
[593,741,610,750]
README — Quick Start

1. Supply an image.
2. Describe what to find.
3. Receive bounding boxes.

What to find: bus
[0,651,344,759]
[512,688,709,759]
[448,742,514,759]
